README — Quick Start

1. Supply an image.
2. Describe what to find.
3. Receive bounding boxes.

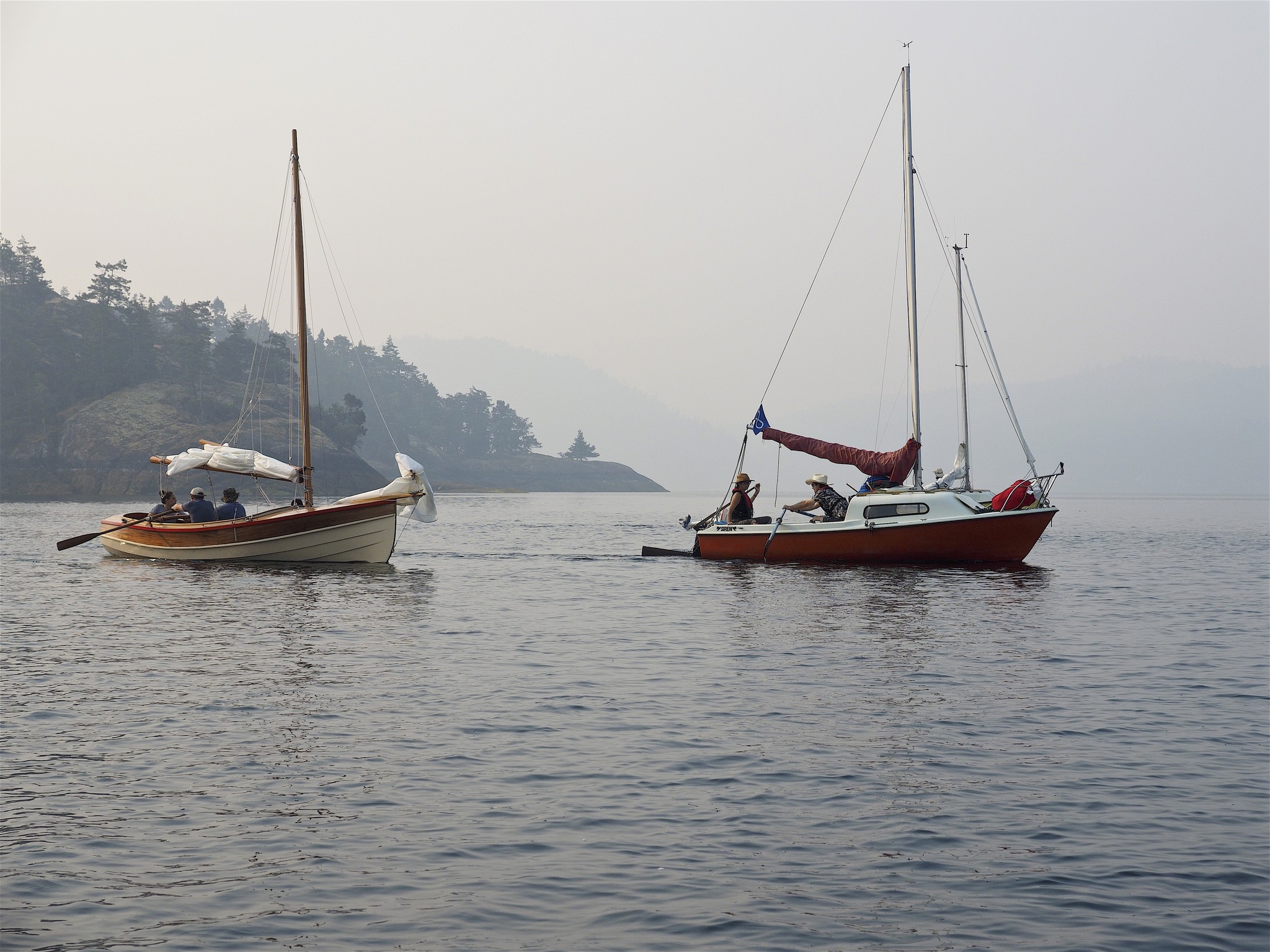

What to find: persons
[214,487,246,521]
[932,468,944,480]
[726,474,772,525]
[147,491,187,523]
[782,474,849,523]
[173,487,216,523]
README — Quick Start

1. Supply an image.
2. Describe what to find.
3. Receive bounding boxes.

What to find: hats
[732,473,755,483]
[933,468,944,477]
[220,487,239,502]
[805,474,834,485]
[188,487,205,496]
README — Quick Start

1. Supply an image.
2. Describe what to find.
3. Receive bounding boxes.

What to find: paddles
[56,508,178,551]
[151,514,190,524]
[763,507,787,558]
[790,508,822,519]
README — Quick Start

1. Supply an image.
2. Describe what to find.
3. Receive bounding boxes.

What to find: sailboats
[634,54,1056,569]
[91,124,439,567]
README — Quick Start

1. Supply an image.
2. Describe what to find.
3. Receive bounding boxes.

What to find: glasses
[811,483,817,487]
[741,481,751,484]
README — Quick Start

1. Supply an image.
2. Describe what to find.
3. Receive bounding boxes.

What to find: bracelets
[797,509,800,512]
[754,494,757,497]
